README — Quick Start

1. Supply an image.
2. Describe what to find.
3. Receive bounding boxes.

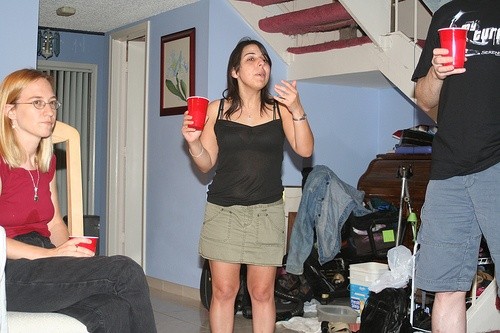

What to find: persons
[181,36,315,333]
[0,68,158,333]
[411,0,500,333]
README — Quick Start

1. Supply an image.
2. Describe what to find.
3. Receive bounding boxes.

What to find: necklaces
[23,155,40,201]
[239,97,261,120]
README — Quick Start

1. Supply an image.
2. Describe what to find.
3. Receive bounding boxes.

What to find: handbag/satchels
[359,284,412,333]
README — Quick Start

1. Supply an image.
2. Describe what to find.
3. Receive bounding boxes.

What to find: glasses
[6,100,61,110]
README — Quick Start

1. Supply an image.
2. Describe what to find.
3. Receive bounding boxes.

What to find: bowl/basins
[316,305,359,323]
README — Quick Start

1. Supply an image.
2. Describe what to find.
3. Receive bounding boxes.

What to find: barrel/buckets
[348,262,389,310]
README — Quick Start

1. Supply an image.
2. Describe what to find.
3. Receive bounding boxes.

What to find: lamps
[37,28,60,59]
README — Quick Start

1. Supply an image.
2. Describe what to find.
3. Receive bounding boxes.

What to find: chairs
[346,211,406,261]
[0,121,89,333]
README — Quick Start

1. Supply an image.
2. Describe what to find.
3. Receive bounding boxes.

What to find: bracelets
[188,142,204,158]
[292,111,307,121]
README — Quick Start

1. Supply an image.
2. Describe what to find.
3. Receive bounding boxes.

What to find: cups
[437,28,467,69]
[69,236,99,254]
[186,96,209,131]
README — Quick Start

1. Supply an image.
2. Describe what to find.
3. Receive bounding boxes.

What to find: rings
[75,245,78,251]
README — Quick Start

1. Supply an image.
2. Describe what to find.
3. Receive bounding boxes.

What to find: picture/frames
[160,26,196,117]
